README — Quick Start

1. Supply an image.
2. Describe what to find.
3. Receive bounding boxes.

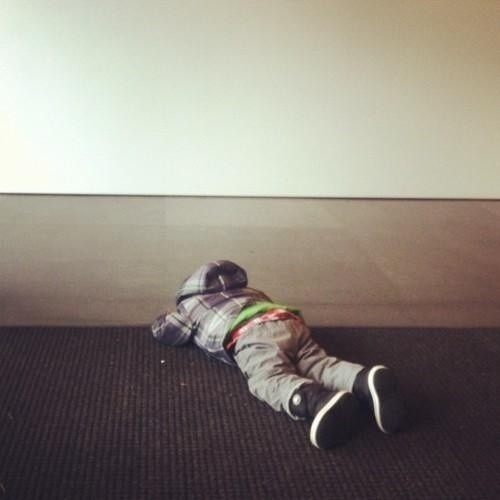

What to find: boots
[289,383,352,450]
[352,365,401,434]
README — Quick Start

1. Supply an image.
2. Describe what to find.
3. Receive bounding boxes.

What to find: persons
[151,260,404,450]
